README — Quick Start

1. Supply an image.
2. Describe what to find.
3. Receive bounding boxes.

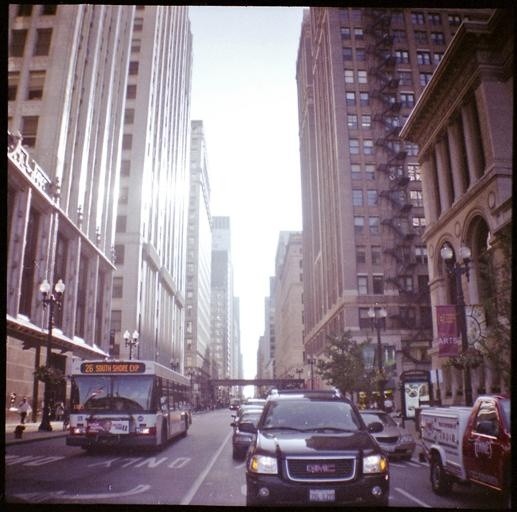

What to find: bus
[65,359,192,450]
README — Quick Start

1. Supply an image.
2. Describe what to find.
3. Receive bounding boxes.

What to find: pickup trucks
[421,397,511,509]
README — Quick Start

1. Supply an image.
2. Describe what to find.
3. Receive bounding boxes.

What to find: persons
[54,400,64,421]
[17,397,30,424]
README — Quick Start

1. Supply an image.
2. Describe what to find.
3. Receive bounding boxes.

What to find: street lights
[368,306,387,410]
[307,354,316,389]
[187,368,196,393]
[440,244,472,407]
[124,330,139,360]
[170,358,179,371]
[38,279,65,430]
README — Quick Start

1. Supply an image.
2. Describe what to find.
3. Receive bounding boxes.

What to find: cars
[229,389,416,506]
